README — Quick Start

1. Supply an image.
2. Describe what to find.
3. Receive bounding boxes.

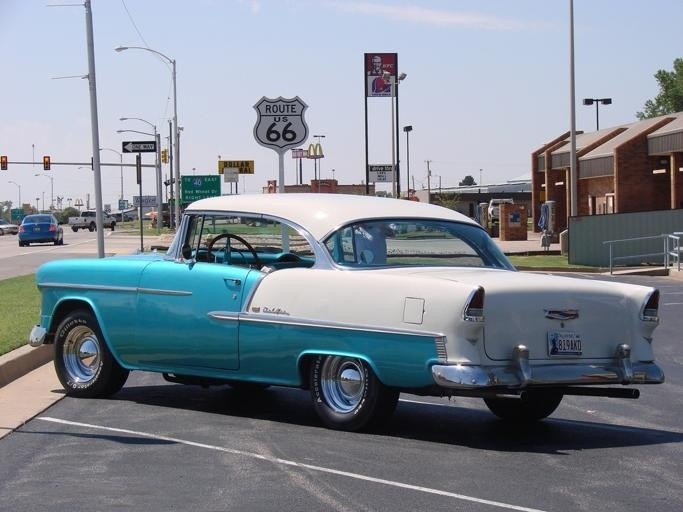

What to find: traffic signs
[252,95,307,150]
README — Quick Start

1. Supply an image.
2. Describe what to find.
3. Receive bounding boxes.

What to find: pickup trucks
[68,209,116,231]
[488,199,514,222]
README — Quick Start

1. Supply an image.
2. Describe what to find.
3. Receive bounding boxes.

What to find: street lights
[79,166,93,170]
[116,117,163,232]
[9,180,21,209]
[115,46,180,229]
[584,98,613,132]
[36,174,54,210]
[99,148,123,200]
[315,134,325,195]
[382,71,406,199]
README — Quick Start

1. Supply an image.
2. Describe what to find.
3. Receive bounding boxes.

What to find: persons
[366,55,394,76]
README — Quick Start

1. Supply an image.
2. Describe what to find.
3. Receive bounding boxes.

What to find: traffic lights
[1,156,7,170]
[44,156,49,169]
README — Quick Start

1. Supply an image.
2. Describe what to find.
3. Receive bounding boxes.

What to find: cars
[0,218,18,235]
[19,214,63,246]
[32,193,665,432]
